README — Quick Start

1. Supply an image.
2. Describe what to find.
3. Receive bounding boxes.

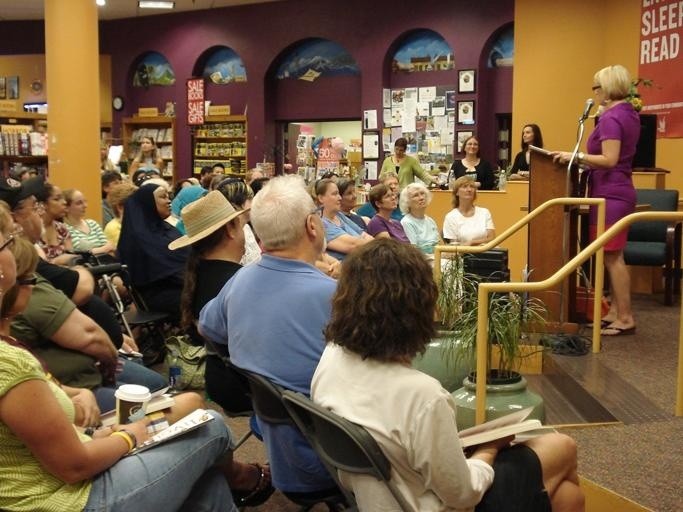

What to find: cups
[115,384,152,424]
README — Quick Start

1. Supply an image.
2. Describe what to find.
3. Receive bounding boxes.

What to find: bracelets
[521,171,524,176]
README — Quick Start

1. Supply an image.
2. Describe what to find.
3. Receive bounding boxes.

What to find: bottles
[169,368,180,386]
[498,169,507,190]
[449,170,456,190]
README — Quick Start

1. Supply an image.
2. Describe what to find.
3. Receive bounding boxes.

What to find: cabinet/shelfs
[122,116,175,182]
[0,110,49,180]
[192,115,248,178]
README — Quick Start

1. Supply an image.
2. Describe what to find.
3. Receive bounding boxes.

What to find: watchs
[578,152,584,163]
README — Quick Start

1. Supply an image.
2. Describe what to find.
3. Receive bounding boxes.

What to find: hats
[0,174,45,211]
[167,190,250,251]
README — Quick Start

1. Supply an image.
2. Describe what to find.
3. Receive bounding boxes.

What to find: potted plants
[441,283,560,431]
[414,251,477,392]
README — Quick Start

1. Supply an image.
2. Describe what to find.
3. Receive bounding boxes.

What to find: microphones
[580,98,594,123]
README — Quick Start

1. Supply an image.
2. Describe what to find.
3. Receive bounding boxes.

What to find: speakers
[462,248,510,321]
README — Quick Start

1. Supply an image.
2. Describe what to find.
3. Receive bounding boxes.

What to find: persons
[447,136,495,189]
[102,140,463,416]
[197,176,339,507]
[311,239,586,512]
[1,168,276,512]
[443,176,496,246]
[547,64,641,336]
[507,124,543,181]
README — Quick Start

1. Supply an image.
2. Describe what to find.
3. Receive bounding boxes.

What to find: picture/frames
[457,100,475,125]
[362,130,380,161]
[456,130,474,154]
[458,69,476,93]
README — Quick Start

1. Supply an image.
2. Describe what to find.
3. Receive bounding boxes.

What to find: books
[0,123,246,177]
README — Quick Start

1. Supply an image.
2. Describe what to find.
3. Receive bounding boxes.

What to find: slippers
[586,320,635,336]
[231,462,275,507]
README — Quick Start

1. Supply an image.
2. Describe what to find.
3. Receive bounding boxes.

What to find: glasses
[17,273,38,286]
[0,234,17,254]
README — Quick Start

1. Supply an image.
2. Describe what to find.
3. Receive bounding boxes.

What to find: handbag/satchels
[161,336,210,390]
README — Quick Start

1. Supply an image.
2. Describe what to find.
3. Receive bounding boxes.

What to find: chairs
[621,188,680,307]
